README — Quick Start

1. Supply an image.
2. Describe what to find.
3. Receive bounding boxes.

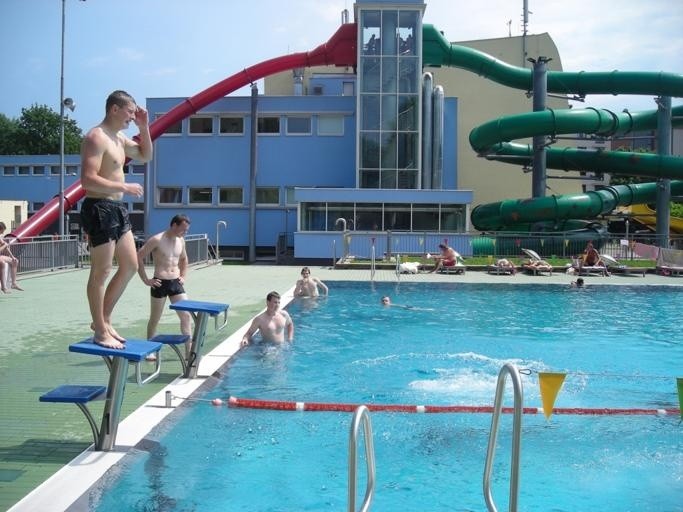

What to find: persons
[240,291,293,348]
[527,260,551,269]
[494,258,514,268]
[582,243,609,276]
[572,277,584,288]
[0,221,23,294]
[293,267,328,297]
[78,89,154,351]
[381,295,390,305]
[137,214,192,361]
[428,244,456,274]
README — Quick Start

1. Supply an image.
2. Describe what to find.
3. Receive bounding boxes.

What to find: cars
[134,235,145,248]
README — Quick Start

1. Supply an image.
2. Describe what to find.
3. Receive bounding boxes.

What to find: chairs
[401,256,683,276]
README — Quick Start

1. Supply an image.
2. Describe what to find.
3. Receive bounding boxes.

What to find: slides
[422,25,682,257]
[0,25,356,258]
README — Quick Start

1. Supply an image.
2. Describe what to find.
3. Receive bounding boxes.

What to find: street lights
[56,97,75,268]
